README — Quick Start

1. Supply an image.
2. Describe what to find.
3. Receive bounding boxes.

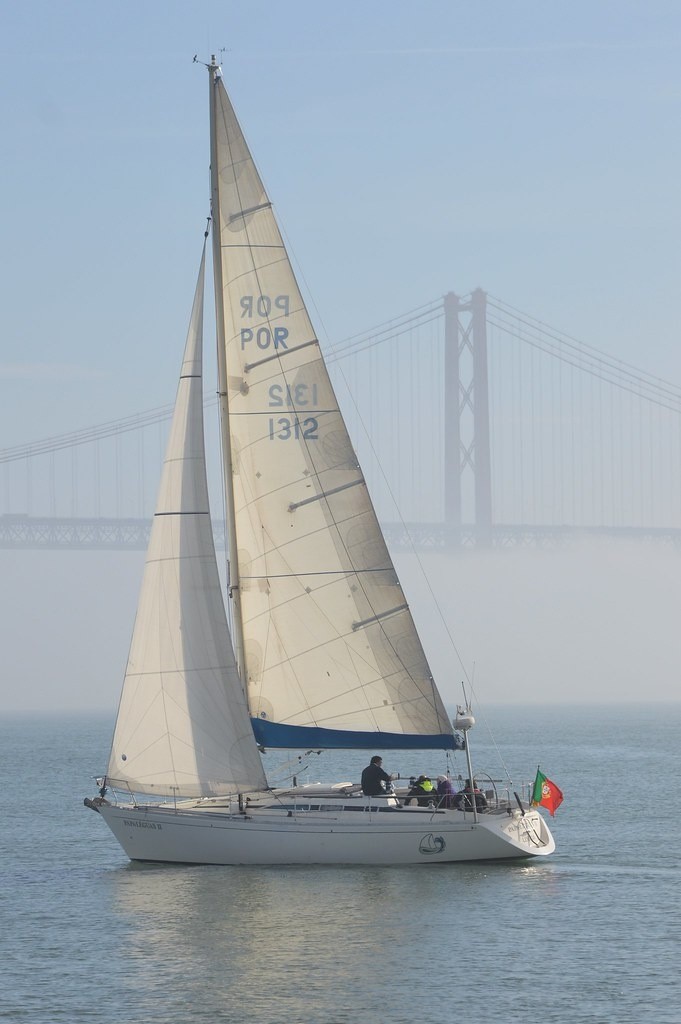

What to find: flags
[531,770,564,818]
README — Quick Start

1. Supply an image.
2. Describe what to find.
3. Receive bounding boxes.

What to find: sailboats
[84,50,557,865]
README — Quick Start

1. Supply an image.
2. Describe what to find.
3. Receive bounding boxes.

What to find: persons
[404,775,438,807]
[452,779,488,813]
[361,756,400,804]
[436,775,457,809]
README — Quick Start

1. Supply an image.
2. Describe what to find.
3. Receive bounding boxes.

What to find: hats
[438,775,446,783]
